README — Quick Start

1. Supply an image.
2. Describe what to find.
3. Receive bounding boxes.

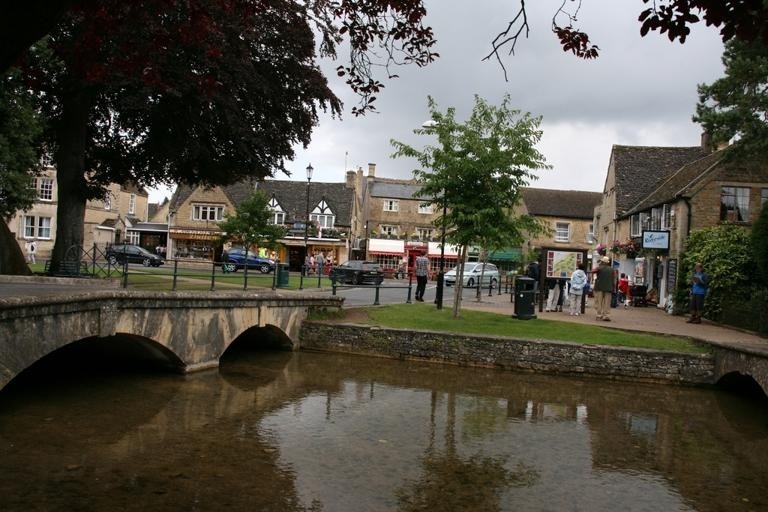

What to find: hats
[600,256,610,263]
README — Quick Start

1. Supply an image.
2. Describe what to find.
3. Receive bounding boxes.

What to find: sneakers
[415,295,424,301]
[570,311,581,316]
[546,306,562,312]
[686,317,701,323]
[596,317,611,322]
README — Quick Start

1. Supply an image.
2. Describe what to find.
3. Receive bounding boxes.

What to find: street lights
[305,164,313,257]
[422,118,448,307]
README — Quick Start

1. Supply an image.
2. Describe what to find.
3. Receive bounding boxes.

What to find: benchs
[45,259,97,278]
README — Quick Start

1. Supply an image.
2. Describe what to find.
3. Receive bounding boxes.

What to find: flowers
[595,238,641,256]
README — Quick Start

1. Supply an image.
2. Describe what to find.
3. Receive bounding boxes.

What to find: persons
[567,263,588,316]
[302,250,338,276]
[414,251,432,302]
[593,256,616,323]
[221,249,231,274]
[527,256,570,313]
[269,250,278,263]
[685,263,708,324]
[618,273,628,304]
[155,244,167,259]
[25,239,38,265]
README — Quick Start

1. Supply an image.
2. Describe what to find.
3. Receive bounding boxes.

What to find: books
[687,275,705,285]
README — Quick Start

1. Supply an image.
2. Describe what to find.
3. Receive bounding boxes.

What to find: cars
[222,248,276,274]
[329,260,385,285]
[444,262,499,289]
[104,244,166,268]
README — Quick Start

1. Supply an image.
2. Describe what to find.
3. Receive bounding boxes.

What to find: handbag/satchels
[584,282,590,293]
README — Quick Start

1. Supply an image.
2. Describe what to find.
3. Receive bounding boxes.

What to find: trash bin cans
[276,264,289,287]
[513,277,536,320]
